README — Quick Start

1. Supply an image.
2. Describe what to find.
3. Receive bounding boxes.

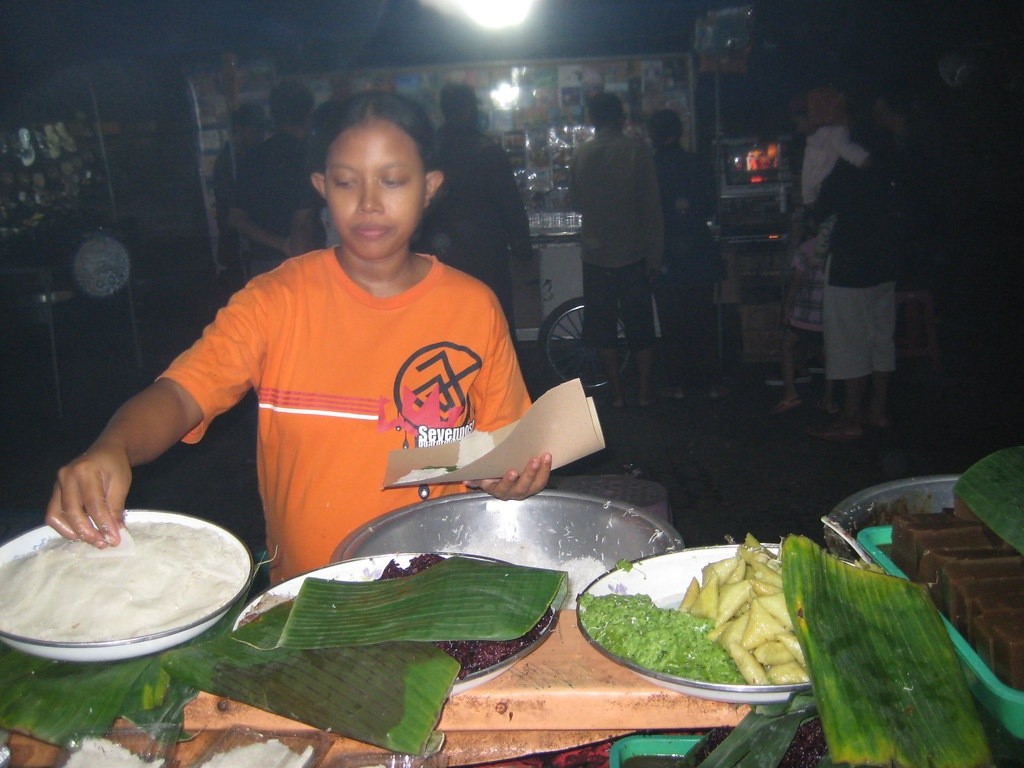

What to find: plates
[236,551,558,700]
[0,510,253,662]
[575,543,810,703]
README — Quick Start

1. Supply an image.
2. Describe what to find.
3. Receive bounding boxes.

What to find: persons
[45,91,553,584]
[568,92,729,406]
[764,71,932,442]
[211,81,530,347]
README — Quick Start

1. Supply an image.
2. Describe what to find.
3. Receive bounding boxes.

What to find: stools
[893,273,942,360]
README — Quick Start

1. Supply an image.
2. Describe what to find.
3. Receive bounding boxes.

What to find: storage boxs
[741,284,817,364]
[857,525,1024,739]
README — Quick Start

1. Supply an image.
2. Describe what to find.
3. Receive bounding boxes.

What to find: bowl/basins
[825,475,961,563]
[330,488,686,567]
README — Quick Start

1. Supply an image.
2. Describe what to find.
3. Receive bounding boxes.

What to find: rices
[366,553,554,678]
[431,501,666,610]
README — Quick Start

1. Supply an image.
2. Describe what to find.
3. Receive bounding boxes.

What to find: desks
[0,216,143,418]
[0,609,752,768]
